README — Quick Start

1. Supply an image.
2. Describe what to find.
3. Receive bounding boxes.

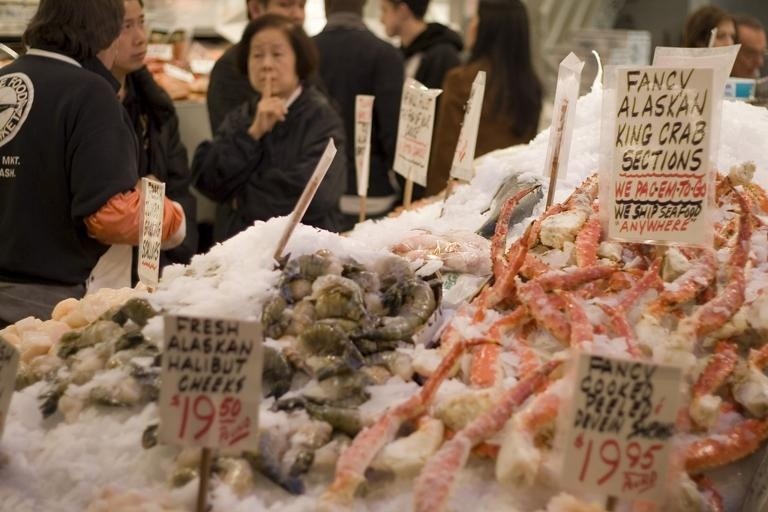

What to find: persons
[730,14,768,80]
[189,14,349,247]
[426,1,545,197]
[378,0,466,92]
[308,0,426,230]
[207,0,343,139]
[0,1,189,331]
[111,1,200,290]
[683,7,740,49]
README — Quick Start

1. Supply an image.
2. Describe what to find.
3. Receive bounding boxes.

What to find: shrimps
[32,243,444,497]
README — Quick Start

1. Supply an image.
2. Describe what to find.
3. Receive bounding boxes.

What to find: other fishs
[475,169,546,240]
